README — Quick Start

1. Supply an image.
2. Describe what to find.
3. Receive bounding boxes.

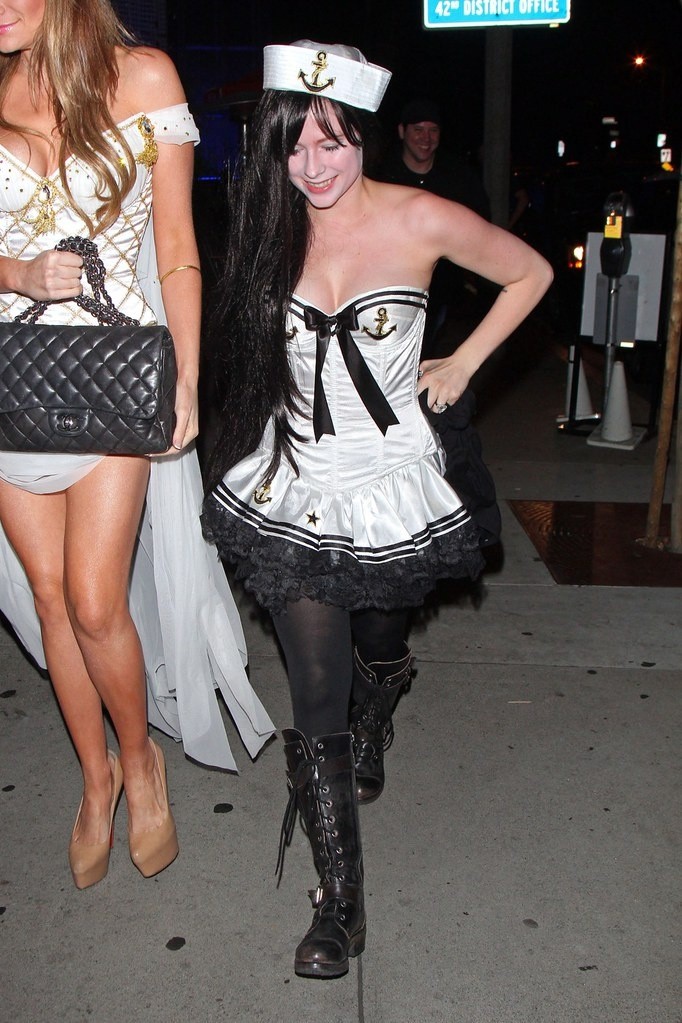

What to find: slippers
[353,639,413,805]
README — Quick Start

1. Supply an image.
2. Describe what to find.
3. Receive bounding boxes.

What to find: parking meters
[597,190,635,425]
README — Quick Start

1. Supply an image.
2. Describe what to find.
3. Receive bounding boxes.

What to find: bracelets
[161,264,201,282]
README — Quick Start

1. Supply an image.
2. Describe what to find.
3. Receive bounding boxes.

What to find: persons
[367,105,504,575]
[198,34,553,978]
[0,0,276,889]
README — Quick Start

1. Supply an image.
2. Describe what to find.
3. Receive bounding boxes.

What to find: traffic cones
[585,361,649,452]
[555,345,601,423]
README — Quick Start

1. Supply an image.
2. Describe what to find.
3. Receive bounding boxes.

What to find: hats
[402,100,441,127]
[262,40,392,112]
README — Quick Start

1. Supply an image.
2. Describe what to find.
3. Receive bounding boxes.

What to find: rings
[418,370,423,377]
[435,402,447,412]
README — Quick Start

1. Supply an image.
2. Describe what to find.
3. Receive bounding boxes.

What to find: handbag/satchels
[0,236,176,455]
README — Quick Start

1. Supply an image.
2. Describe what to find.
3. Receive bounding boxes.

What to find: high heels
[123,736,179,877]
[69,749,124,890]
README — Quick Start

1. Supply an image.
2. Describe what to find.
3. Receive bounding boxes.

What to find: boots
[273,728,367,976]
